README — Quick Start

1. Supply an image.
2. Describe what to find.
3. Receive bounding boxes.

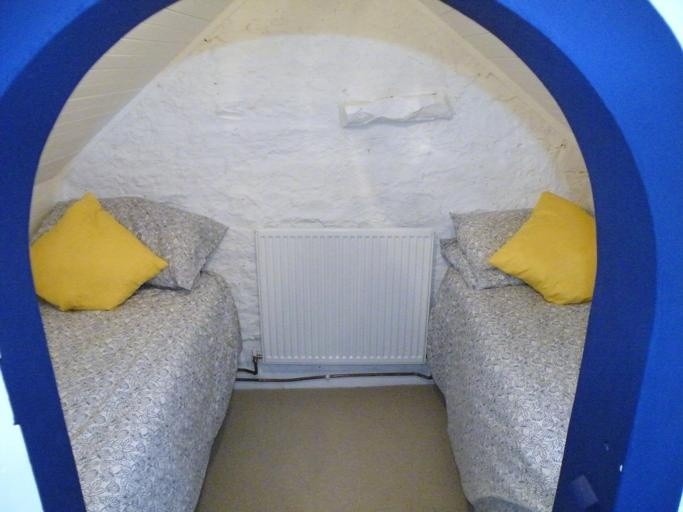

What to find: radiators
[253,227,434,365]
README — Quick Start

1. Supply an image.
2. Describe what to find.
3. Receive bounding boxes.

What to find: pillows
[29,191,228,310]
[439,190,597,305]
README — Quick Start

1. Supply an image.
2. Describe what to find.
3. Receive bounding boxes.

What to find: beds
[33,268,242,512]
[424,254,596,512]
